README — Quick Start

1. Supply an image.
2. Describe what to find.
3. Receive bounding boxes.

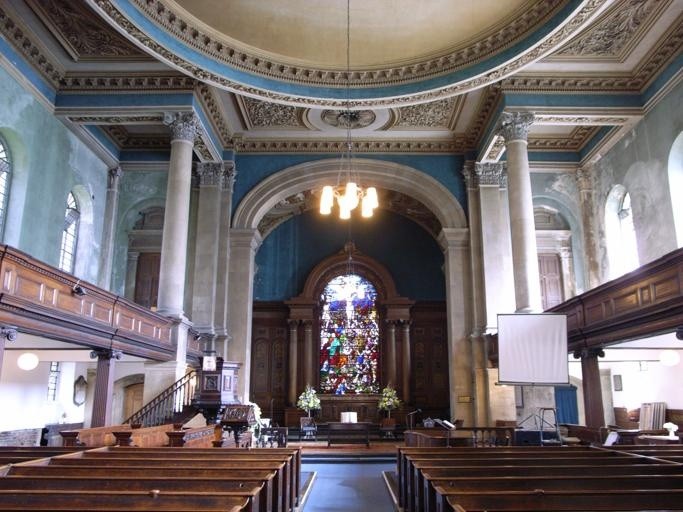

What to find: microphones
[408,409,422,416]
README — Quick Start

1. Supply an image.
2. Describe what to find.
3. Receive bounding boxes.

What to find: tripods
[518,385,556,428]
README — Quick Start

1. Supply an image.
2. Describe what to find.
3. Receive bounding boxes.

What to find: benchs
[380,440,682,511]
[1,444,302,512]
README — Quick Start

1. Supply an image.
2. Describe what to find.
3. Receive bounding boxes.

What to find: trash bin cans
[40,428,49,445]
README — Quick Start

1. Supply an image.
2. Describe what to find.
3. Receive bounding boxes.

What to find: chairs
[380,419,396,439]
[298,417,318,443]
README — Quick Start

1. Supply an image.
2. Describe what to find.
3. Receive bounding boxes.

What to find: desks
[325,422,374,448]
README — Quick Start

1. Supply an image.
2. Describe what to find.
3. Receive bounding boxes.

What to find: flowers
[297,386,321,418]
[377,385,403,418]
